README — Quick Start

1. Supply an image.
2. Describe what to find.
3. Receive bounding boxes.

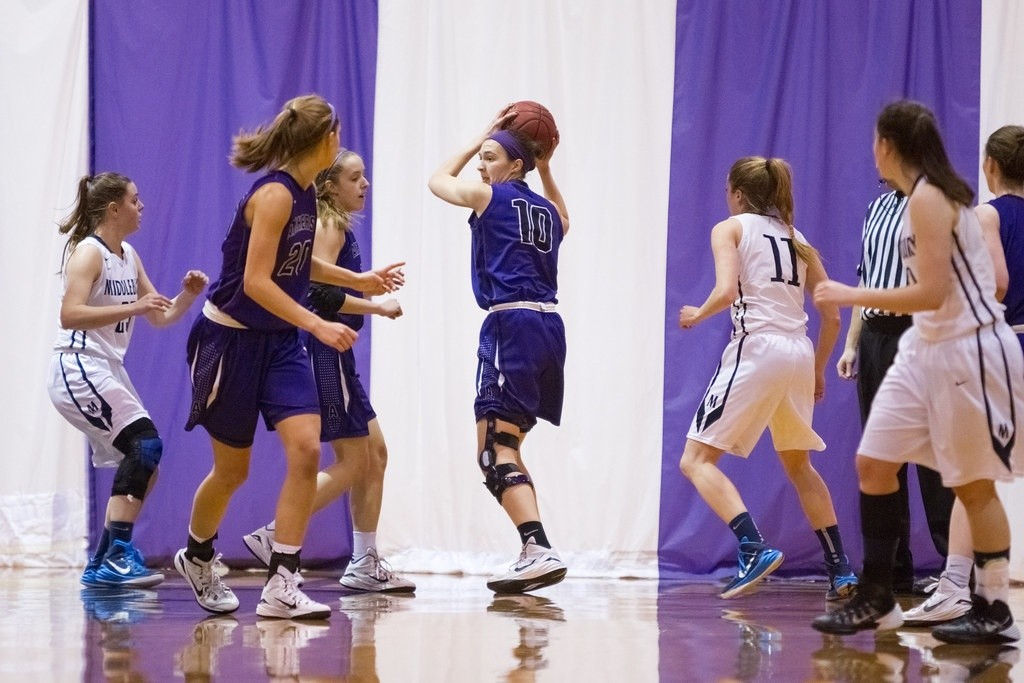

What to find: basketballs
[502,102,555,157]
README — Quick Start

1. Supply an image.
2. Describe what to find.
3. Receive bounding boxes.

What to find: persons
[678,155,861,601]
[243,148,417,594]
[172,91,407,621]
[42,169,211,590]
[427,102,570,594]
[811,101,1023,645]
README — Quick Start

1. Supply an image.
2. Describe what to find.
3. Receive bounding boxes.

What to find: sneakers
[340,592,415,621]
[173,545,241,613]
[811,572,905,635]
[810,645,909,683]
[931,592,1022,643]
[826,569,860,601]
[485,593,565,623]
[338,547,417,592]
[486,535,567,593]
[172,615,238,678]
[95,537,166,585]
[720,536,785,599]
[919,643,1021,683]
[81,588,164,624]
[256,617,332,647]
[80,555,155,590]
[256,564,333,619]
[720,608,782,653]
[901,570,974,622]
[241,524,305,587]
[912,574,941,595]
